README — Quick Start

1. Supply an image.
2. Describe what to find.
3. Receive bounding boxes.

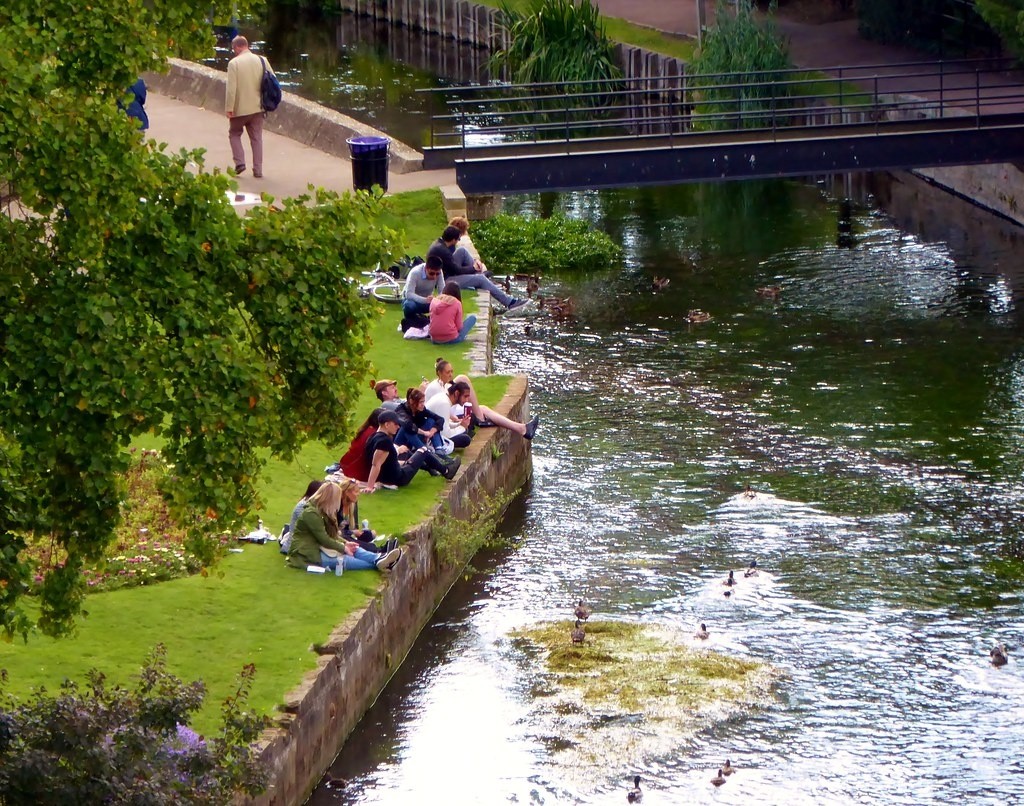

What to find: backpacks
[256,56,281,111]
[404,256,425,280]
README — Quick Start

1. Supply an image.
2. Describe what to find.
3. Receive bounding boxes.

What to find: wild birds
[721,570,737,599]
[504,273,572,319]
[627,776,644,804]
[990,642,1009,666]
[742,483,756,500]
[694,623,709,639]
[711,759,734,786]
[755,284,779,300]
[575,599,590,620]
[571,620,587,644]
[689,244,704,262]
[685,308,709,324]
[744,560,758,578]
[653,277,670,289]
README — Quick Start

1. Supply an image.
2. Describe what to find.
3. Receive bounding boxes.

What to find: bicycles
[346,261,407,304]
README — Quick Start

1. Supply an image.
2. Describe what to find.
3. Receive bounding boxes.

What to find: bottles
[257,515,263,530]
[237,537,266,545]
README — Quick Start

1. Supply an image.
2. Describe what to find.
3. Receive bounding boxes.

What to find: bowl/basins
[345,136,392,158]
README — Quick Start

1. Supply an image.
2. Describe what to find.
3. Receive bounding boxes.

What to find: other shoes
[235,164,246,174]
[482,270,493,278]
[253,168,262,177]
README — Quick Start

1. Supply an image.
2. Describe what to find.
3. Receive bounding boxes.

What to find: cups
[335,564,343,575]
[464,402,473,418]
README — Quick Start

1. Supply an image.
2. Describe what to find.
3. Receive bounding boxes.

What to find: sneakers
[392,538,398,550]
[476,415,496,427]
[442,455,461,480]
[435,448,454,461]
[523,414,539,440]
[375,548,400,569]
[379,539,393,556]
[427,467,441,476]
[464,286,476,290]
[510,297,529,311]
[386,548,403,572]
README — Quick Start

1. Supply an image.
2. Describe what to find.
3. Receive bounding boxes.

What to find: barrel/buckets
[350,154,390,193]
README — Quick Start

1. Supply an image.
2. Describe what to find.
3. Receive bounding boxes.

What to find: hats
[374,379,397,393]
[378,410,405,426]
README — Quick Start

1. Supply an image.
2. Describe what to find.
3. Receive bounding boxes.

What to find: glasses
[457,237,460,241]
[427,269,441,276]
[345,479,355,491]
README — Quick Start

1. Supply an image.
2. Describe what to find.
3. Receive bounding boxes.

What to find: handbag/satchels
[426,433,454,454]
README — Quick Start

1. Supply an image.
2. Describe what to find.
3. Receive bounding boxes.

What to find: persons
[225,36,276,179]
[117,77,149,141]
[279,357,539,571]
[402,217,531,347]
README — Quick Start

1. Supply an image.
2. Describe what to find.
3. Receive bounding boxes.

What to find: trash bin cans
[346,136,392,194]
